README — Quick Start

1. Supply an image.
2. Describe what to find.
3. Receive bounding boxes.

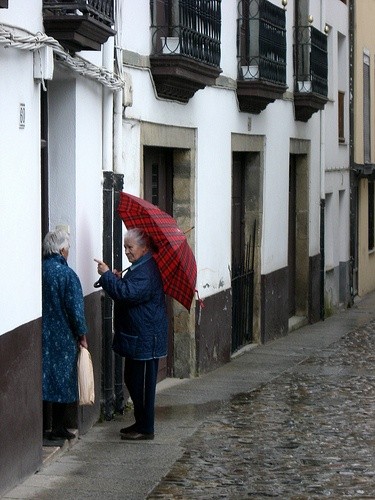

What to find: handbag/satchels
[77,346,95,405]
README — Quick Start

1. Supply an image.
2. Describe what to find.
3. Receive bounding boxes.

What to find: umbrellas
[94,191,197,314]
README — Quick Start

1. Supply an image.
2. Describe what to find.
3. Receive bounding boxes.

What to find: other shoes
[44,427,76,441]
[120,422,155,440]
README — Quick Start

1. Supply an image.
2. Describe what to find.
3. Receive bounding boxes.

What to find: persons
[94,228,169,439]
[42,229,88,439]
